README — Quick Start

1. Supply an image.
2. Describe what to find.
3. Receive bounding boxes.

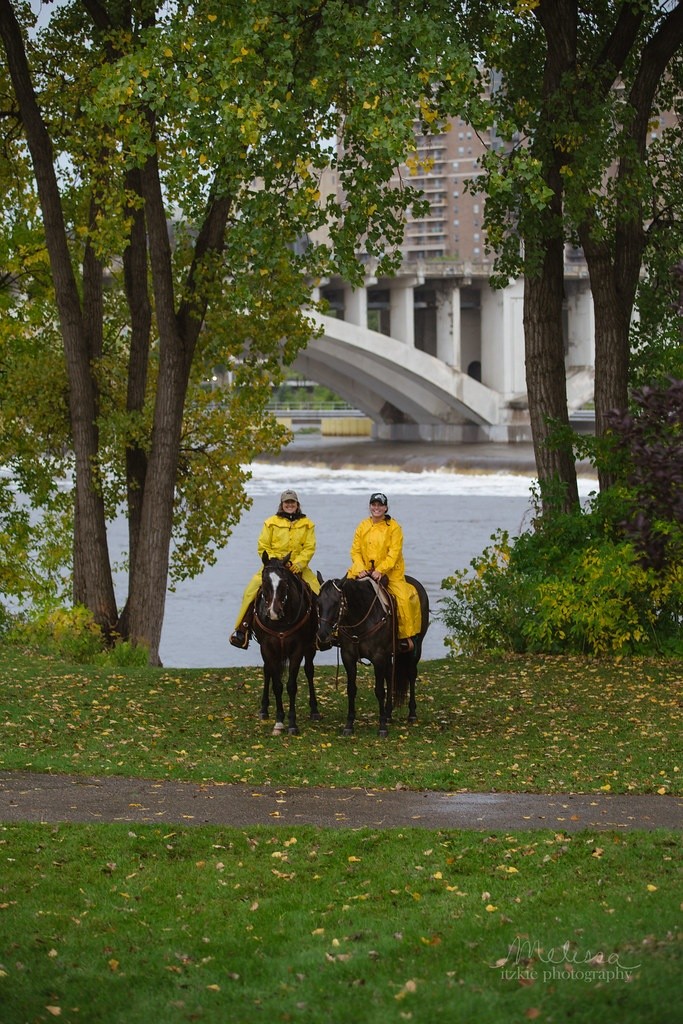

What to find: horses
[314,570,430,739]
[252,549,319,736]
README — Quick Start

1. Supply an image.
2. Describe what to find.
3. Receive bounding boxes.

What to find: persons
[229,490,321,650]
[346,493,421,652]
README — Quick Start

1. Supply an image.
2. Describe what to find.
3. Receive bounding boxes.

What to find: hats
[370,493,387,505]
[281,490,298,502]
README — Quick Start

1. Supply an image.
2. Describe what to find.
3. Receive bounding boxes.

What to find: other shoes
[229,630,250,649]
[400,638,416,656]
[331,637,341,646]
